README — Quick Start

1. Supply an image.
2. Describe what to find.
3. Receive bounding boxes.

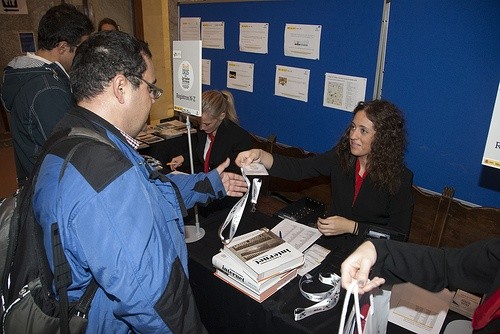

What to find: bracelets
[352,222,356,234]
[355,227,358,234]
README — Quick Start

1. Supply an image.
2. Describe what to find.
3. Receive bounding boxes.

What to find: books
[212,252,286,290]
[134,119,195,149]
[222,227,305,284]
[212,265,298,303]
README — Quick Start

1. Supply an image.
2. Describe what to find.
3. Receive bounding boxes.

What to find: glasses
[124,74,163,98]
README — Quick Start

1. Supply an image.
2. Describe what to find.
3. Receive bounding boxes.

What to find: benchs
[247,132,500,249]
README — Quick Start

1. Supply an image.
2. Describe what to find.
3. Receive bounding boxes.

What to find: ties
[203,132,214,172]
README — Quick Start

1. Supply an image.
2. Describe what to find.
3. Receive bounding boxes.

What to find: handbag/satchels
[340,278,389,334]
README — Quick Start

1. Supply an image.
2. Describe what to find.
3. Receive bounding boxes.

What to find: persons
[98,18,121,32]
[170,90,261,216]
[0,3,95,190]
[236,100,414,242]
[341,237,500,334]
[32,30,249,334]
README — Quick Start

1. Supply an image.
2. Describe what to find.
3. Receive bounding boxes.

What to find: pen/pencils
[165,162,177,166]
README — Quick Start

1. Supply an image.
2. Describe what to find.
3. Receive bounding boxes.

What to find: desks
[162,167,484,334]
[138,133,203,173]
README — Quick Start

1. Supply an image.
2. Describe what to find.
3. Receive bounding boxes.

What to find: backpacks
[0,127,120,334]
